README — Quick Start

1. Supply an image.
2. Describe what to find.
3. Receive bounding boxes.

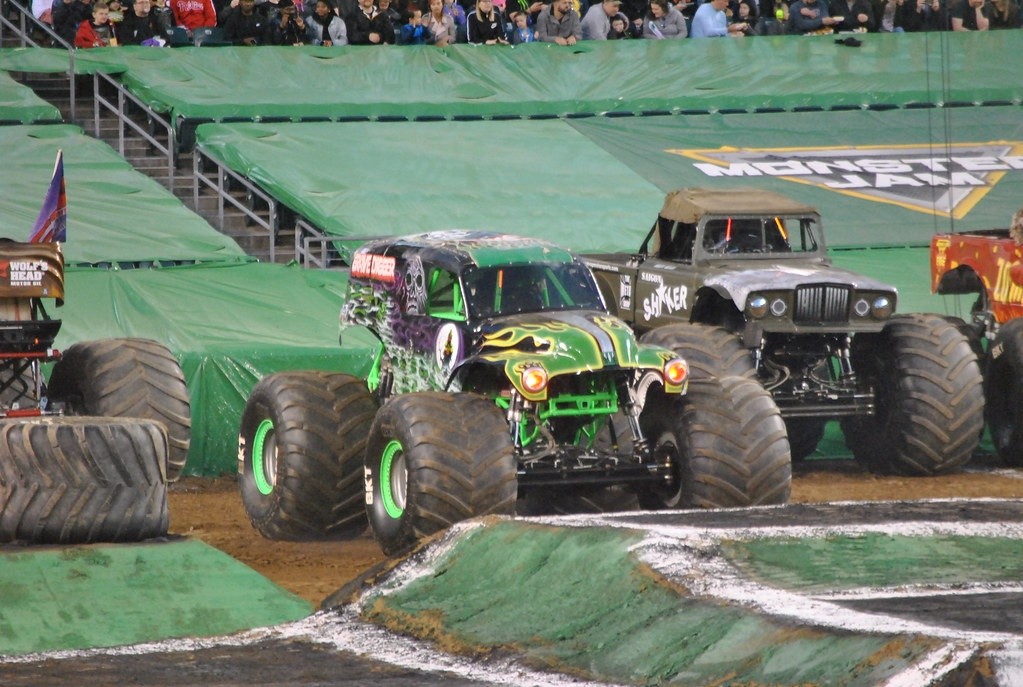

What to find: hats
[604,0,622,4]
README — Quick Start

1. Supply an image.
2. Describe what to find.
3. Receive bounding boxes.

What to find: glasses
[135,1,151,5]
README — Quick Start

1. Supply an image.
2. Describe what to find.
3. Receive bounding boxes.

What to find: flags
[28,150,66,243]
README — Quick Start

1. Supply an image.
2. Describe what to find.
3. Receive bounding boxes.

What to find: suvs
[926,220,1023,467]
[238,228,794,562]
[567,187,989,472]
[0,233,192,489]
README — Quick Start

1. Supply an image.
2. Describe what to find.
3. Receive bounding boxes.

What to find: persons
[4,0,1022,47]
